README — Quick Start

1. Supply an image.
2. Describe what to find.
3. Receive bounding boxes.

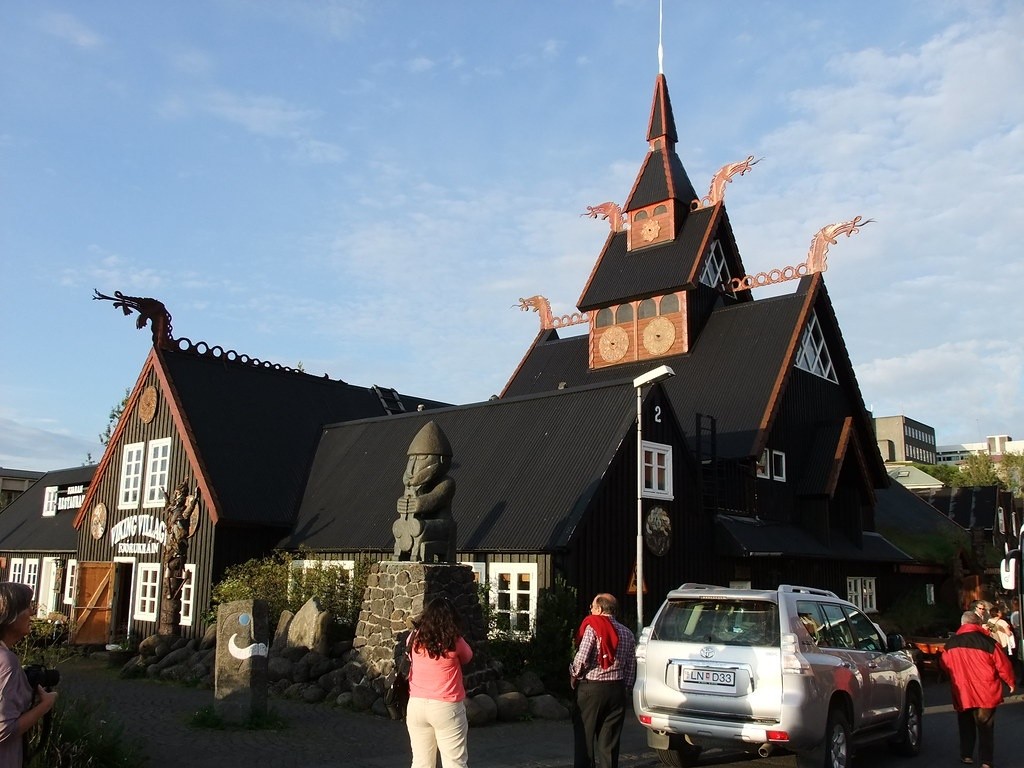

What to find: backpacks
[986,618,1005,645]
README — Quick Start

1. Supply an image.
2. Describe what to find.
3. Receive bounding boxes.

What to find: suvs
[631,582,926,768]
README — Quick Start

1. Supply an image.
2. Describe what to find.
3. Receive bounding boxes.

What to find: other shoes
[981,763,990,768]
[960,757,973,762]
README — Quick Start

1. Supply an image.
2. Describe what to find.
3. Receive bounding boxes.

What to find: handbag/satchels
[383,626,419,707]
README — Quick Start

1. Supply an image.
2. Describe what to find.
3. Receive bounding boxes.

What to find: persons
[158,481,202,569]
[987,607,1016,656]
[975,603,989,631]
[568,593,635,768]
[0,583,57,768]
[393,421,456,563]
[1010,597,1024,688]
[940,611,1016,768]
[406,598,473,768]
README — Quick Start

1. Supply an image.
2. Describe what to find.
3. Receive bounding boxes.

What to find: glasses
[590,604,599,609]
[976,606,986,610]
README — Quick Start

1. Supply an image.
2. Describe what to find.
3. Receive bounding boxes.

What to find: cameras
[21,664,61,690]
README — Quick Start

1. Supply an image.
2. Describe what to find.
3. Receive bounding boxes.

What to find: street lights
[632,364,676,642]
